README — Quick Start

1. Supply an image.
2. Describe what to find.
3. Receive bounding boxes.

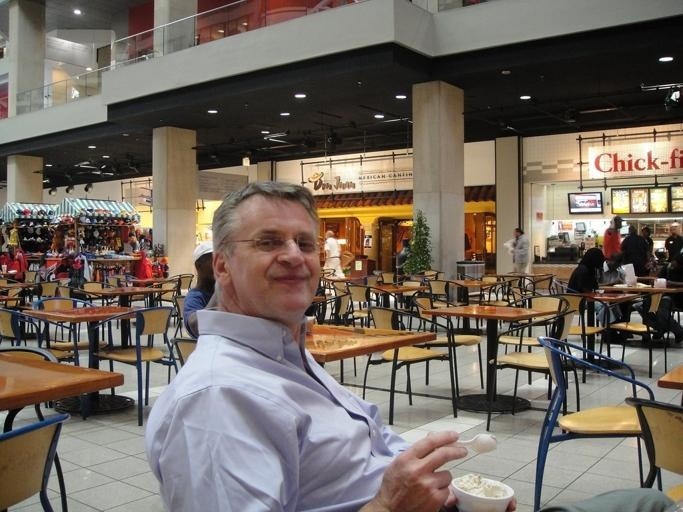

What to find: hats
[192,240,213,264]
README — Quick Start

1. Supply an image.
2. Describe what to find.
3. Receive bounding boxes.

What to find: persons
[320,231,345,287]
[504,228,530,288]
[560,216,683,343]
[130,250,153,287]
[145,181,683,511]
[183,240,217,341]
[128,231,140,252]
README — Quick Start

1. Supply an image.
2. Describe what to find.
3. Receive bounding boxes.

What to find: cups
[625,276,638,286]
[2,265,7,274]
[306,316,316,335]
[655,278,666,288]
[452,478,514,512]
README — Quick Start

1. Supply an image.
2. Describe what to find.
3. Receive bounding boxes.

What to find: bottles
[80,244,111,255]
[472,254,476,261]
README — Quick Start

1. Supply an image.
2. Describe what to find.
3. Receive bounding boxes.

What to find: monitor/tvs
[558,233,569,245]
[576,222,587,232]
[568,192,603,214]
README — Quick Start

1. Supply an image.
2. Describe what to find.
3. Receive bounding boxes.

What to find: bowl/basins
[655,248,669,259]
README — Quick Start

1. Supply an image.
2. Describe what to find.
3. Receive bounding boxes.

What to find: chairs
[1,413,72,512]
[625,397,683,506]
[533,335,662,510]
[1,268,683,427]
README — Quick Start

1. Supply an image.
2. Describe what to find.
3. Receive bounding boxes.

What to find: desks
[1,352,124,434]
[87,258,141,276]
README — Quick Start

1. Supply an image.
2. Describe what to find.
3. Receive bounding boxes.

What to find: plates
[23,252,64,258]
[8,271,17,274]
[595,290,604,293]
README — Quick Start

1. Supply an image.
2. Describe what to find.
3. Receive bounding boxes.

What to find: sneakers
[601,322,683,344]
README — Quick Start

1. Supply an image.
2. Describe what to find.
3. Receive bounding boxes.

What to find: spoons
[429,433,498,453]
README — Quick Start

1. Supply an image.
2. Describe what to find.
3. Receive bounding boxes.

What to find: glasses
[220,236,318,253]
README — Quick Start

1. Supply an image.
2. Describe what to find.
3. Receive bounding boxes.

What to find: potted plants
[399,206,436,282]
